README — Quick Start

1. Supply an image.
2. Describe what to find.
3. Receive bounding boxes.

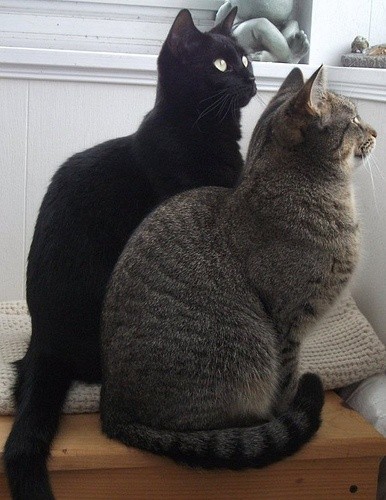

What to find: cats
[97,63,376,470]
[2,5,269,500]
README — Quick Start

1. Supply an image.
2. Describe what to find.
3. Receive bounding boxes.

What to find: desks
[0,389,386,500]
